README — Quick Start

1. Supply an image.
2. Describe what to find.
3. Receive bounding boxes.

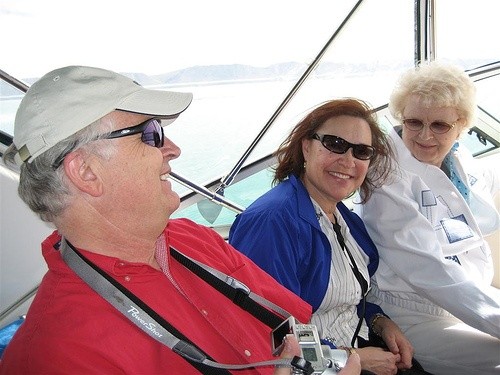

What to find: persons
[228,96,426,375]
[0,66,360,375]
[356,62,500,375]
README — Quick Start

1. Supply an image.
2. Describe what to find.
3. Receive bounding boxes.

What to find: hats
[12,65,193,168]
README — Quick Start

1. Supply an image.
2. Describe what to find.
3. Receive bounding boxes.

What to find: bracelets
[342,345,356,356]
[372,314,392,336]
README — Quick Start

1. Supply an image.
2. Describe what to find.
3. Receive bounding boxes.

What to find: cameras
[272,316,327,375]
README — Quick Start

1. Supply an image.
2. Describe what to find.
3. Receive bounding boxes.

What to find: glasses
[309,132,377,160]
[402,117,458,134]
[51,116,165,172]
